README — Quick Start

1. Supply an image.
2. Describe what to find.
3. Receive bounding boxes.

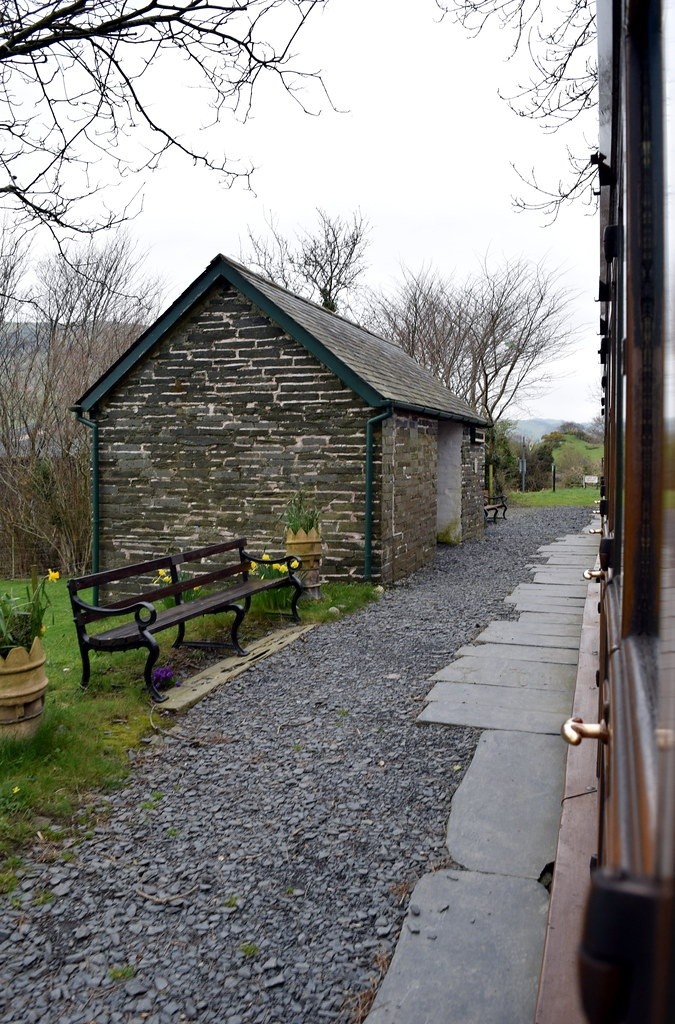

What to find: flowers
[0,568,61,660]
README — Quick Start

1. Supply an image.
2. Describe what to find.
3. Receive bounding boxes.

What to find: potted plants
[285,491,324,571]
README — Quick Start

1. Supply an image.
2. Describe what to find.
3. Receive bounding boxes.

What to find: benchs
[484,494,507,528]
[66,538,303,703]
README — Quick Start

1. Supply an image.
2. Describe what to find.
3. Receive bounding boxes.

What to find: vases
[0,636,50,740]
